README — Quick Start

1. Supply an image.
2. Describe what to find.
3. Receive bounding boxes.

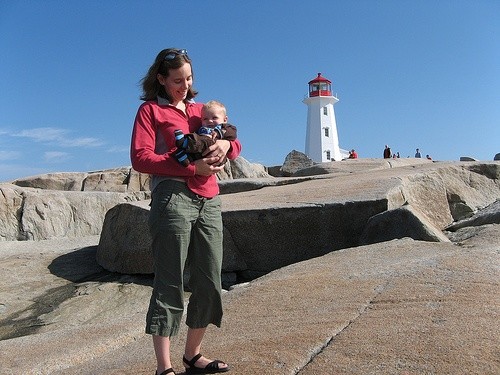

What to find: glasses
[159,49,187,75]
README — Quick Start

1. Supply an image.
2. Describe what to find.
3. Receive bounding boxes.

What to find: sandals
[155,367,175,375]
[182,354,228,372]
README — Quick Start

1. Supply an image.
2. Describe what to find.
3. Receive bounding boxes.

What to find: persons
[329,144,433,160]
[130,47,242,375]
[171,100,237,168]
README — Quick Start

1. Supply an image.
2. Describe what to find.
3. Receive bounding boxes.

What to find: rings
[217,154,221,158]
